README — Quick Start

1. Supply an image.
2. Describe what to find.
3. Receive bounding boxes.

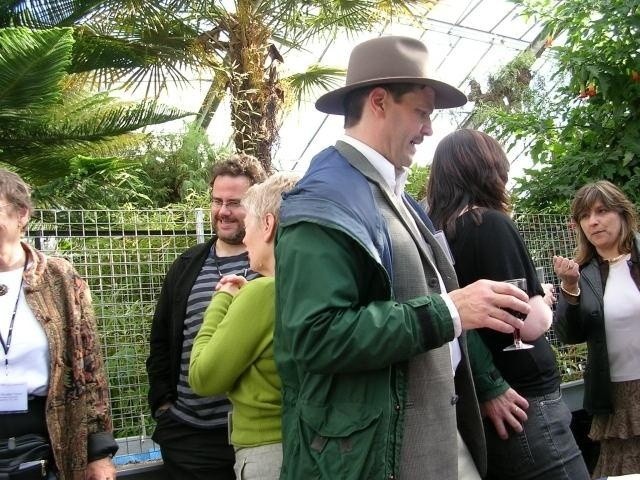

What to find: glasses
[210,197,243,208]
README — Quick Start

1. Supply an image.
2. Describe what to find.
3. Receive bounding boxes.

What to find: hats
[314,35,468,116]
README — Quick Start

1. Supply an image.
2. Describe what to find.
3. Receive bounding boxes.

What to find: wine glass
[498,278,534,351]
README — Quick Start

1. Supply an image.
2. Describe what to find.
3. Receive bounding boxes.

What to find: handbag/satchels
[0,432,55,480]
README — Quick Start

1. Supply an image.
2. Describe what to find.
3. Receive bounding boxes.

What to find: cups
[536,266,545,284]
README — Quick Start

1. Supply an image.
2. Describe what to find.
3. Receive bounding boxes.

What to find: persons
[0,169,120,480]
